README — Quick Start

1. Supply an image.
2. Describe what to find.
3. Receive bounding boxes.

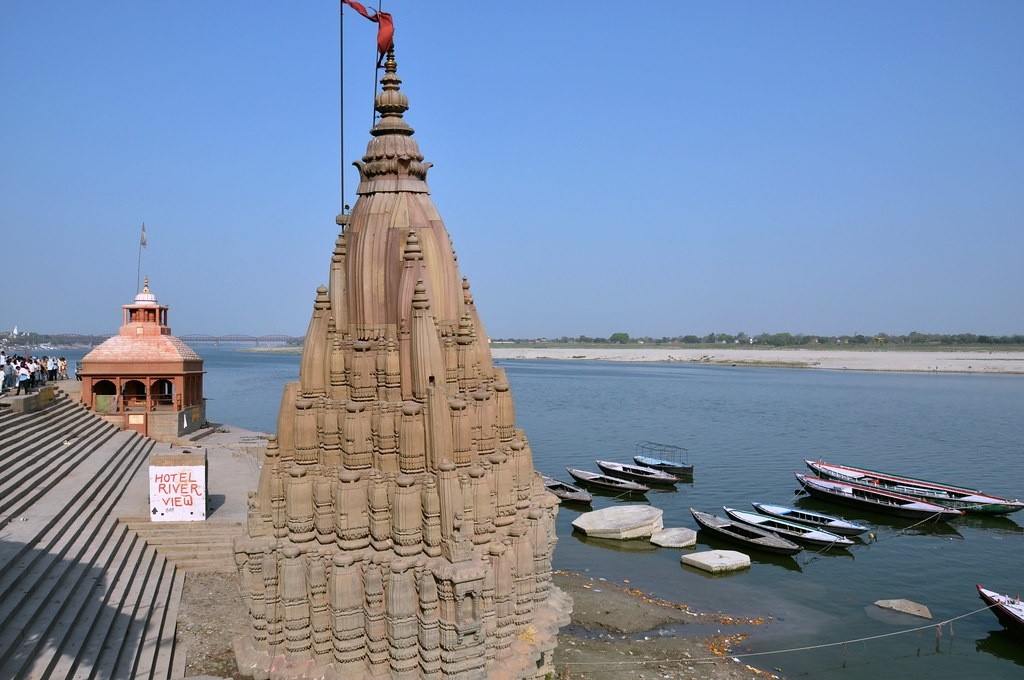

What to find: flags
[141,223,147,248]
[343,0,394,66]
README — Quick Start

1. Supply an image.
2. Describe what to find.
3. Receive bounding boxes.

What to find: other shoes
[26,393,28,394]
[28,386,39,388]
[7,386,17,389]
[15,394,19,395]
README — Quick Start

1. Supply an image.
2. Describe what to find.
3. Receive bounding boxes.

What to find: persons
[0,351,82,395]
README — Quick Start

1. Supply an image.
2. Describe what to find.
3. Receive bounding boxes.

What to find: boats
[723,505,855,549]
[690,507,803,555]
[633,440,694,475]
[794,472,964,519]
[566,467,650,494]
[804,457,1024,514]
[751,502,869,536]
[596,461,680,485]
[537,472,593,502]
[976,584,1024,630]
